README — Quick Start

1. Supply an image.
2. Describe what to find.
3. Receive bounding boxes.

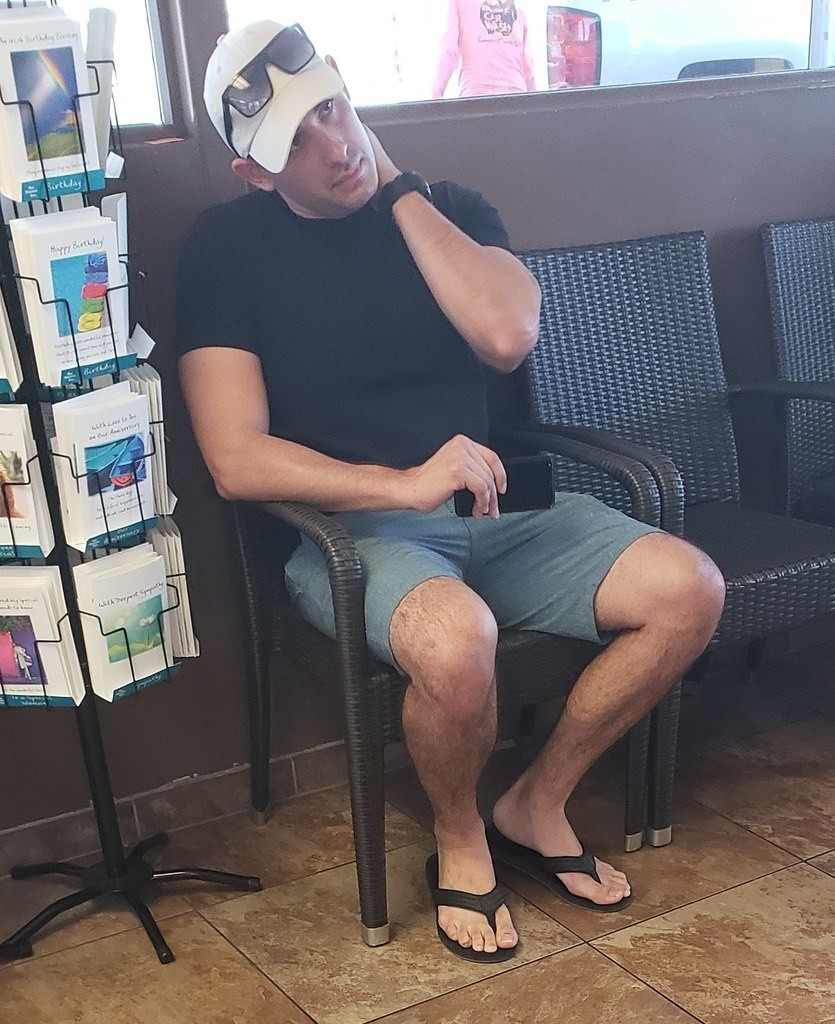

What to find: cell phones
[454,454,555,517]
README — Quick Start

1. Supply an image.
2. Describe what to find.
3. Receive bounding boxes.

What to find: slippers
[425,852,519,963]
[486,822,632,913]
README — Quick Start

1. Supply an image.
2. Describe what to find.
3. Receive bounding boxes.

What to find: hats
[203,20,344,173]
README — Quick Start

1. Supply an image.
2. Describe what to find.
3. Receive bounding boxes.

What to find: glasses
[222,23,315,159]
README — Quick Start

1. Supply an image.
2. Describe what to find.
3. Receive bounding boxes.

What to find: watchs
[380,171,432,220]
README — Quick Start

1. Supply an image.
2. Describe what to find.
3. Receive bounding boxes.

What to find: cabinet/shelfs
[0,59,263,965]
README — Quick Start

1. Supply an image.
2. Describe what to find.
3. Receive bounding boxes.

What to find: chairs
[515,230,835,847]
[761,217,835,525]
[230,430,662,948]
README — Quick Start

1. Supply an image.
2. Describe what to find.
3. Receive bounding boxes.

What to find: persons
[433,0,536,97]
[175,20,725,963]
